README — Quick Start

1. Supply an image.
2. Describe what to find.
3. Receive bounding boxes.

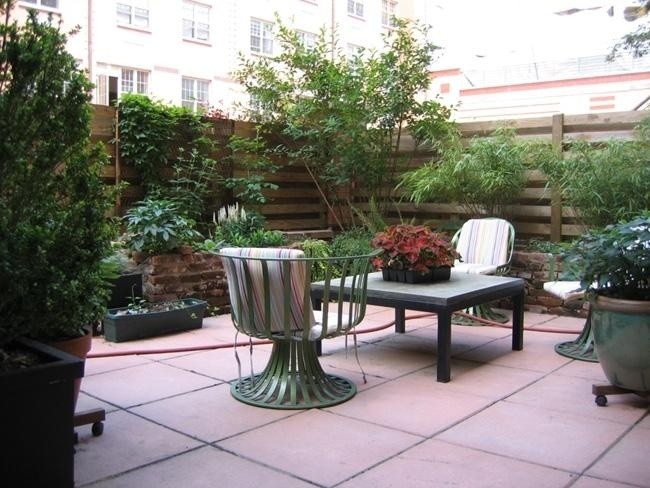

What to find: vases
[379,265,451,283]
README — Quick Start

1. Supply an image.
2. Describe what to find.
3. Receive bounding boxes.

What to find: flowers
[370,223,462,275]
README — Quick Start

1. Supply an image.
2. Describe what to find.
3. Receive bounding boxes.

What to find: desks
[309,271,526,383]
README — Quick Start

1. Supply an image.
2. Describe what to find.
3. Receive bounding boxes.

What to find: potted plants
[1,10,130,443]
[92,270,142,336]
[0,331,84,488]
[103,283,207,344]
[559,214,650,394]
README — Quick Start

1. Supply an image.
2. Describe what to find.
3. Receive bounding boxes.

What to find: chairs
[210,239,385,409]
[450,216,516,326]
[542,226,650,363]
